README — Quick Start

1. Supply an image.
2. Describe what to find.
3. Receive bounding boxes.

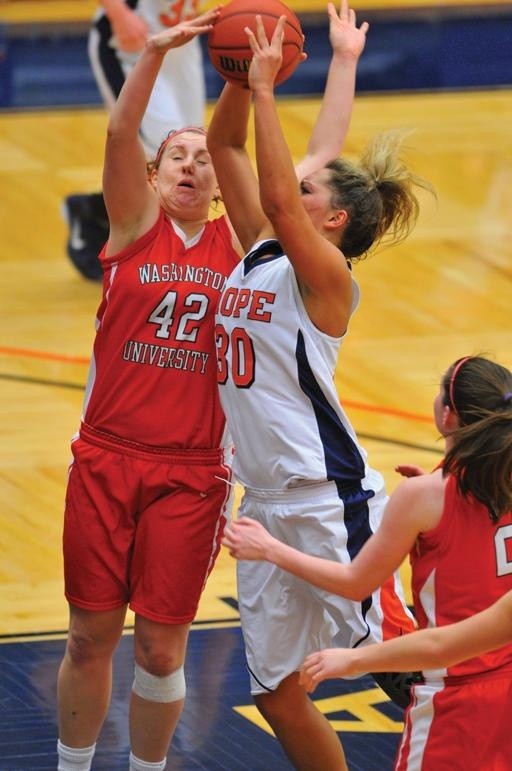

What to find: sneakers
[67,193,103,283]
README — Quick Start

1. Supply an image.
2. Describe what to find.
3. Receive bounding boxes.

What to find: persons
[299,589,511,697]
[222,359,511,771]
[63,1,209,282]
[207,15,422,771]
[53,3,370,770]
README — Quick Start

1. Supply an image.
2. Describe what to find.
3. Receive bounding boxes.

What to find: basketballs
[209,0,303,90]
